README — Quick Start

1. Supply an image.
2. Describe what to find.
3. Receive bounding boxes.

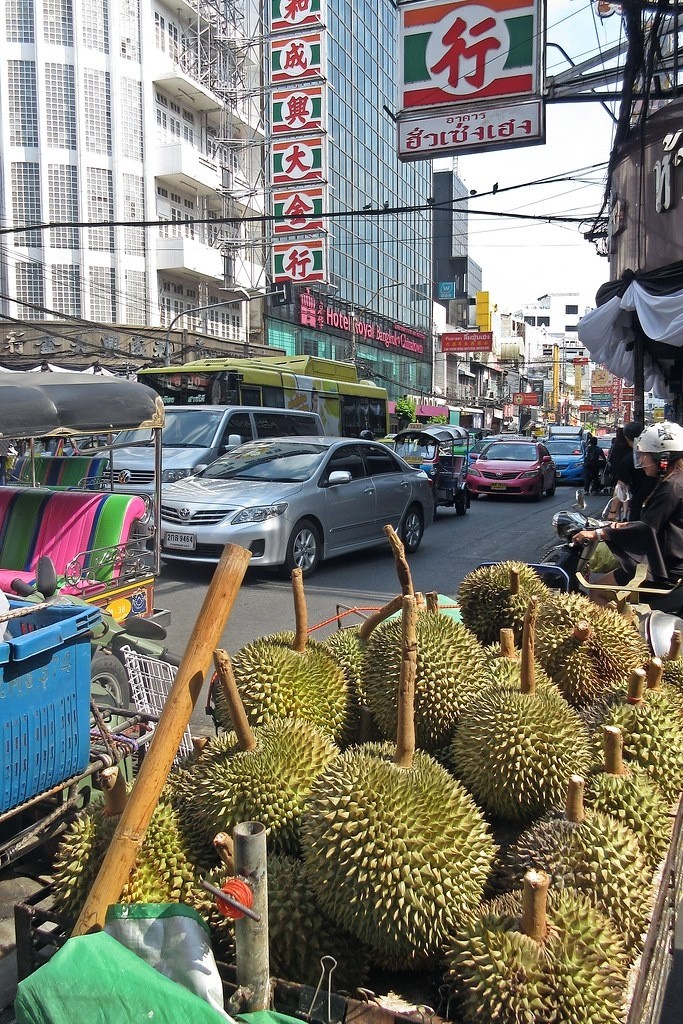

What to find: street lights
[349,281,405,359]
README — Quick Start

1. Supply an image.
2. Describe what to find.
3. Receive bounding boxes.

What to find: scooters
[533,480,683,620]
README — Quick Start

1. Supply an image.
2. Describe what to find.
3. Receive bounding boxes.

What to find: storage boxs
[0,603,100,814]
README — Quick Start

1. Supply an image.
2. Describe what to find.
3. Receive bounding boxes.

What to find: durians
[50,561,683,1024]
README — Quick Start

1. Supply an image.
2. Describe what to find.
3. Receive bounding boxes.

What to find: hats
[623,422,643,441]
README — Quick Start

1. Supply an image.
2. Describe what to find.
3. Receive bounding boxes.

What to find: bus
[137,356,389,444]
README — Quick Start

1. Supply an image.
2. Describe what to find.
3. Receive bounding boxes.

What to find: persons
[310,388,318,413]
[608,427,633,496]
[583,437,607,495]
[571,419,683,614]
[607,422,659,522]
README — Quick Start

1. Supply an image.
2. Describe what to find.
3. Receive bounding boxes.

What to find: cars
[139,436,436,578]
[378,424,616,486]
[465,436,558,501]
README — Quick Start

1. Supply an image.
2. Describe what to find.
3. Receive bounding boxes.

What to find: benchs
[0,485,153,601]
[438,456,465,488]
[10,456,110,491]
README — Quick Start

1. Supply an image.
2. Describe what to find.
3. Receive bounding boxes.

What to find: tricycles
[0,373,172,735]
[394,424,471,515]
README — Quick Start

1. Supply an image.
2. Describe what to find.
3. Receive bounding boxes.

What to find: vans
[91,405,325,504]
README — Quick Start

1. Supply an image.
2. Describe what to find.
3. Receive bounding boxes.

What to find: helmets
[633,421,683,479]
[359,430,374,440]
